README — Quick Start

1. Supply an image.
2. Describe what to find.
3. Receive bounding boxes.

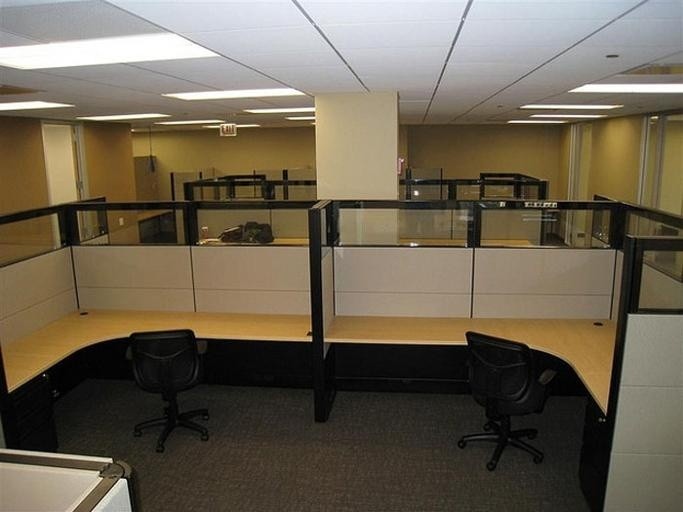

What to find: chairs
[458,330,553,471]
[128,329,211,453]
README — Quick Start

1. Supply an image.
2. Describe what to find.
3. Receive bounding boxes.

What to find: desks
[189,172,551,248]
[2,311,658,510]
